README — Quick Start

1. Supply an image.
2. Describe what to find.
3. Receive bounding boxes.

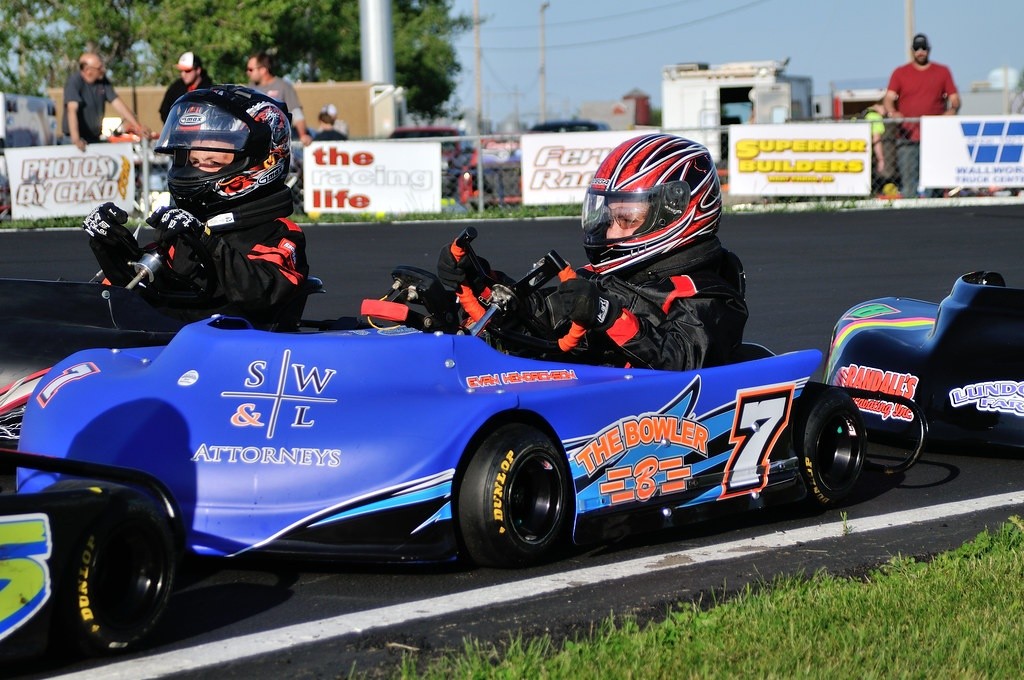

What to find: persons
[80,84,309,333]
[158,52,213,126]
[854,34,962,198]
[246,55,347,147]
[438,133,749,371]
[62,52,151,152]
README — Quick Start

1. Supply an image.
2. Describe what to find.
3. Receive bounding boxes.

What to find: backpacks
[852,110,875,121]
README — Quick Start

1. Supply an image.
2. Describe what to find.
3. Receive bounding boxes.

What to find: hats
[177,53,202,68]
[914,36,927,44]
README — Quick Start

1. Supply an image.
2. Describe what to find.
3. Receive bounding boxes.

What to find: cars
[449,138,523,207]
[385,126,473,198]
[530,120,610,133]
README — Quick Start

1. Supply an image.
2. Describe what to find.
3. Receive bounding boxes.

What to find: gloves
[559,278,619,331]
[158,209,211,249]
[439,241,470,290]
[81,202,128,240]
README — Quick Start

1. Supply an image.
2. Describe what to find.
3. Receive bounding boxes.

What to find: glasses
[178,70,192,73]
[86,65,102,69]
[244,67,261,72]
[601,205,643,230]
[913,46,927,51]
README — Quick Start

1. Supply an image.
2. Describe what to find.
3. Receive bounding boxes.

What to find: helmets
[580,133,722,274]
[154,84,292,224]
